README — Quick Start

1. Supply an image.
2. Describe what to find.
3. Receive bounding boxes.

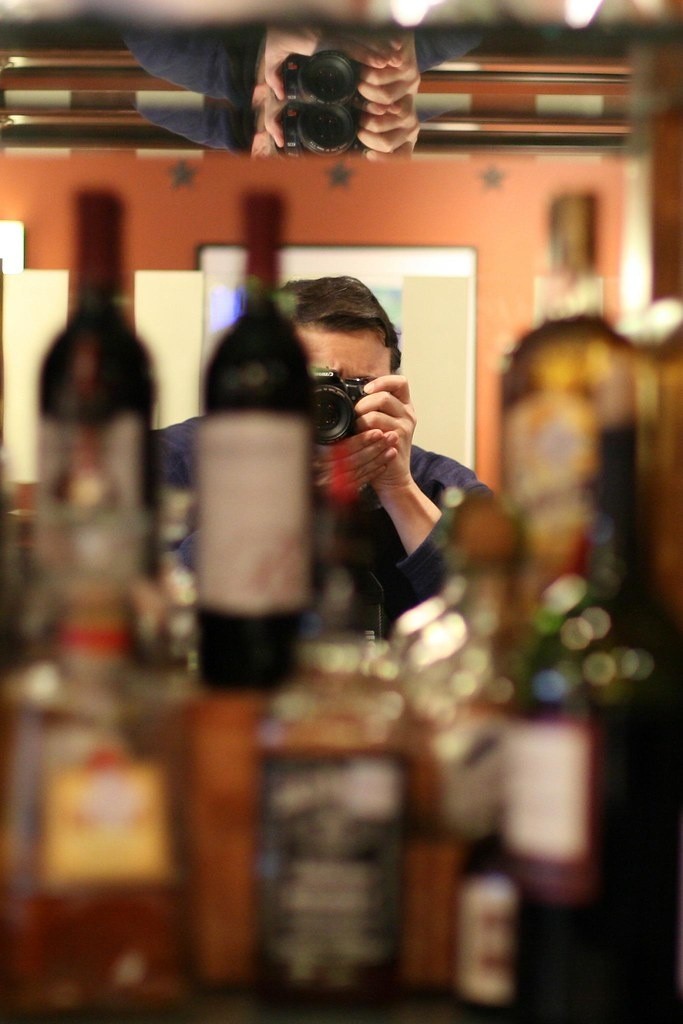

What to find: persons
[148,276,501,647]
[115,0,496,160]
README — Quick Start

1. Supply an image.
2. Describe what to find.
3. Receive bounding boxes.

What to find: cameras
[268,51,370,161]
[311,367,377,445]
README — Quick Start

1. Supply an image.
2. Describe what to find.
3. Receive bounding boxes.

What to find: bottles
[38,189,156,643]
[511,574,683,1024]
[512,595,598,905]
[498,186,640,658]
[197,188,317,685]
[457,832,518,1001]
[0,617,177,1013]
